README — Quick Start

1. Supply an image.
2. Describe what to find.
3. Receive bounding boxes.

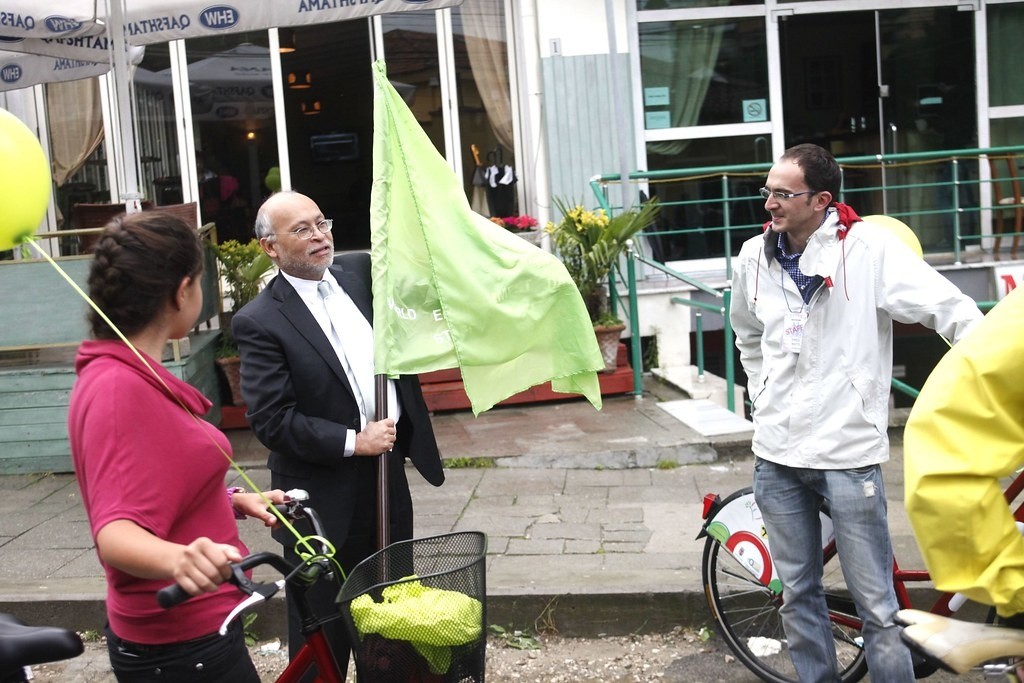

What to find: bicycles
[0,488,492,682]
[693,455,1024,683]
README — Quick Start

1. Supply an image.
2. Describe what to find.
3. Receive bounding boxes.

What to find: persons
[195,149,220,204]
[727,143,994,683]
[901,277,1023,630]
[66,208,293,683]
[227,187,446,683]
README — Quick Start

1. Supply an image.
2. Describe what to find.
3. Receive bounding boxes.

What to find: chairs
[141,202,197,231]
[71,203,126,255]
[988,154,1024,260]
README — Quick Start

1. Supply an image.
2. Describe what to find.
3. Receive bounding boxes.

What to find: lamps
[279,28,321,114]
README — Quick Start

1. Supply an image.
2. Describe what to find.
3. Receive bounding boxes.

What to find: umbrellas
[129,39,291,121]
[468,143,516,218]
[0,1,476,214]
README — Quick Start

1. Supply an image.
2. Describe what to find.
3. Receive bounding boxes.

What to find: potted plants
[591,310,626,374]
[200,236,277,407]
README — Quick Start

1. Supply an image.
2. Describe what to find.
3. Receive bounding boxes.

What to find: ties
[317,282,377,424]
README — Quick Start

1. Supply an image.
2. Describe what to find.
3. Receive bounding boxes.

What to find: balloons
[0,102,51,253]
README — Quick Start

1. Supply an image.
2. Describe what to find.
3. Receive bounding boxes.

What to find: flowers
[539,194,662,296]
[489,215,539,234]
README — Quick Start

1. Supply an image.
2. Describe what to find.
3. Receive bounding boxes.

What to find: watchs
[226,486,247,522]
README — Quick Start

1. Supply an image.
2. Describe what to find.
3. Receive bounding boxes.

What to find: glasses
[268,219,333,240]
[759,187,816,201]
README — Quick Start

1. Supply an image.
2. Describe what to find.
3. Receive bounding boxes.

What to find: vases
[512,230,538,246]
[585,287,608,321]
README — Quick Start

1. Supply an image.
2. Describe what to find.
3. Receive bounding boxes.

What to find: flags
[369,54,610,420]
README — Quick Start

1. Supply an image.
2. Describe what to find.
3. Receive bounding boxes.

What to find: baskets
[334,531,489,683]
